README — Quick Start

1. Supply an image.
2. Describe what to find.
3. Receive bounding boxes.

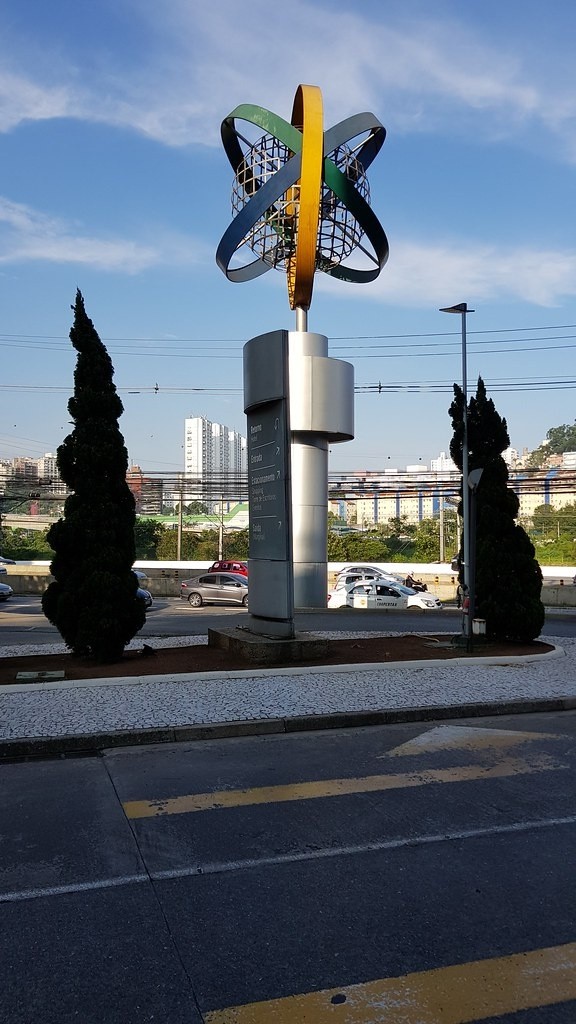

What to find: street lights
[439,303,476,642]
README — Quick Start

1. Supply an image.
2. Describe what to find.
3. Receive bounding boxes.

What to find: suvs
[208,561,248,577]
[335,566,405,584]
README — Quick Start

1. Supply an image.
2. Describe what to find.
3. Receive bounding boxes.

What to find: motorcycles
[412,580,432,595]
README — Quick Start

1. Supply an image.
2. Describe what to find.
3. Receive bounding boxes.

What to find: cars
[327,581,443,613]
[138,588,153,610]
[0,556,16,565]
[181,572,249,608]
[0,582,13,601]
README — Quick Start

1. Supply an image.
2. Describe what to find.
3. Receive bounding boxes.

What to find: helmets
[408,570,414,577]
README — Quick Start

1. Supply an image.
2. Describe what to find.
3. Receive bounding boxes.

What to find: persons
[403,568,423,590]
[354,577,359,581]
[382,586,391,596]
